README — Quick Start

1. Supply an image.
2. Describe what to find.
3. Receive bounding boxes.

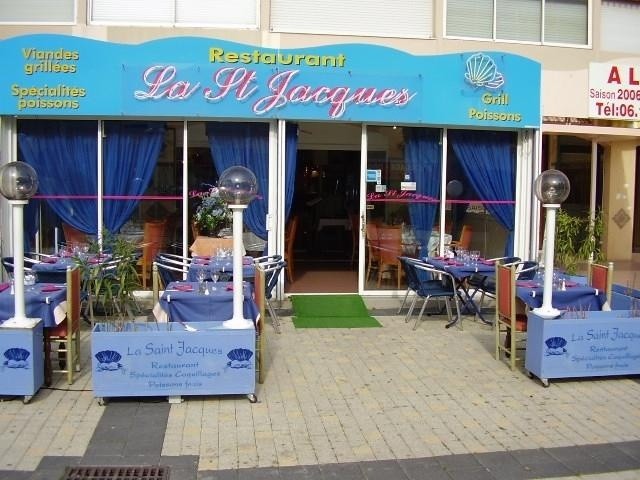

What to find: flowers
[191,183,232,231]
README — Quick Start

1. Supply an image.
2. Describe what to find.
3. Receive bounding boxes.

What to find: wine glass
[216,244,234,271]
[49,242,90,268]
[196,268,219,295]
[23,269,41,295]
[444,246,481,267]
[534,265,560,289]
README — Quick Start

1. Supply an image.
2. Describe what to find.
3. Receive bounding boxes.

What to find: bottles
[9,282,15,296]
[558,278,568,291]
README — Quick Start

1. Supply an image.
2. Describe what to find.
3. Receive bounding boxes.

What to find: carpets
[291,295,384,328]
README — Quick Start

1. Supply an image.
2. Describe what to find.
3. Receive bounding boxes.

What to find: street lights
[447,180,463,245]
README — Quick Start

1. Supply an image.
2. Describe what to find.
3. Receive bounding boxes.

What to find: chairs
[347,208,359,264]
[43,263,83,384]
[2,216,298,325]
[215,217,299,384]
[366,223,614,372]
